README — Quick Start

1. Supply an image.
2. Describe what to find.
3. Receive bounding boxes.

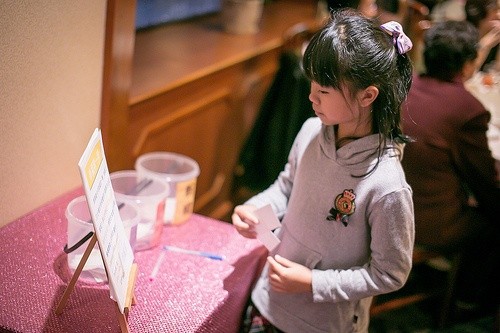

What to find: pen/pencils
[149,249,165,281]
[164,244,222,260]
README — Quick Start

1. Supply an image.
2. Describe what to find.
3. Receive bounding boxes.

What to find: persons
[231,8,415,333]
[323,0,500,322]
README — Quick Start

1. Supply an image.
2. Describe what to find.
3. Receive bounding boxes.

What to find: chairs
[235,47,463,333]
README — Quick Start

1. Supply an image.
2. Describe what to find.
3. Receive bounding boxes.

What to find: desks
[0,184,269,333]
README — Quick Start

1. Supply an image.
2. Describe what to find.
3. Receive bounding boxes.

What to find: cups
[107,168,170,251]
[62,194,142,284]
[134,151,201,227]
